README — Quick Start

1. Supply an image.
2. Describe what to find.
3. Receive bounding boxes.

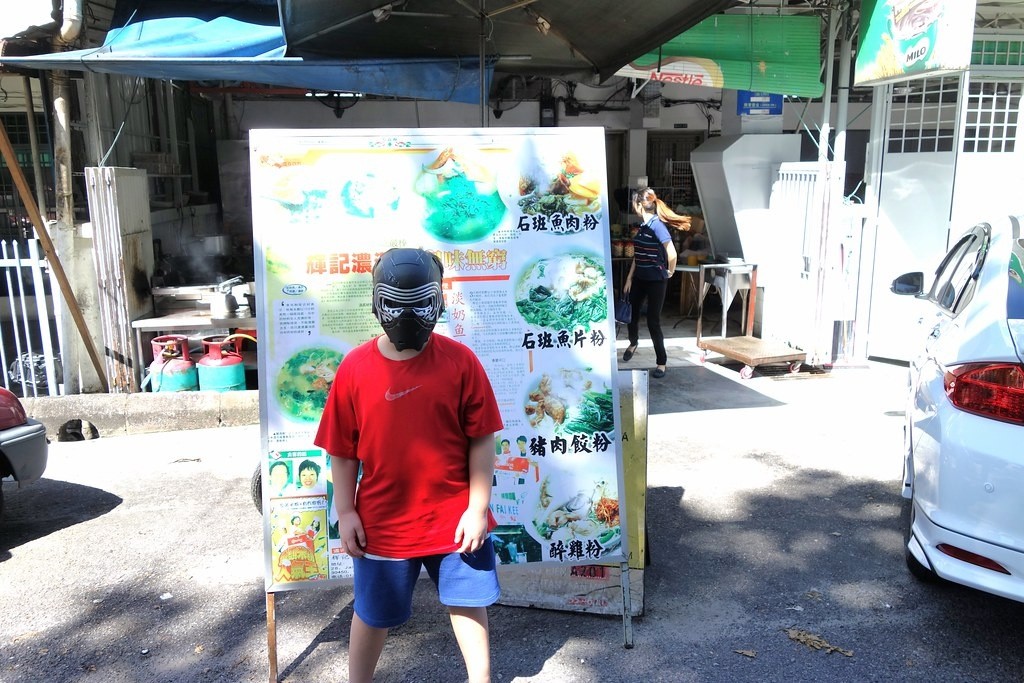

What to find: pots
[192,235,235,256]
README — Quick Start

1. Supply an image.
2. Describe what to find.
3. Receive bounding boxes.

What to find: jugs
[209,290,239,313]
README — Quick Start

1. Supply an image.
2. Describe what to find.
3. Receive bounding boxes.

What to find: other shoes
[623,344,637,361]
[654,364,666,377]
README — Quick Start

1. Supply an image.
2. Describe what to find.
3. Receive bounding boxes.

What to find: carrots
[598,498,619,526]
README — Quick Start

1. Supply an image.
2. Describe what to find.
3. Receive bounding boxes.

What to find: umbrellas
[278,0,742,130]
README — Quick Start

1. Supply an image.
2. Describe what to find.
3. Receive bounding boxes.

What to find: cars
[888,210,1024,609]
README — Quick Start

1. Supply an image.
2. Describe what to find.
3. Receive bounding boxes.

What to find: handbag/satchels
[615,290,631,324]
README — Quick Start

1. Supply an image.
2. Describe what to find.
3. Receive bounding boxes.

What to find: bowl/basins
[247,296,255,315]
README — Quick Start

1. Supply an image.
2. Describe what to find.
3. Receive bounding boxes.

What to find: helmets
[370,248,448,352]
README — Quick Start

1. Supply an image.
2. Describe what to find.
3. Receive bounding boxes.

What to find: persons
[516,435,528,457]
[269,460,293,498]
[623,187,692,378]
[497,538,517,565]
[313,248,504,682]
[277,513,322,553]
[296,459,324,495]
[500,439,512,456]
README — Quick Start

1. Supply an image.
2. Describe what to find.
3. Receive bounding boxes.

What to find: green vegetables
[516,285,608,334]
[279,349,343,416]
[566,385,611,435]
[517,192,568,216]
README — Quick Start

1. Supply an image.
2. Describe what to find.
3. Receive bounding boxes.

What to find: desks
[132,312,250,393]
[674,264,717,328]
